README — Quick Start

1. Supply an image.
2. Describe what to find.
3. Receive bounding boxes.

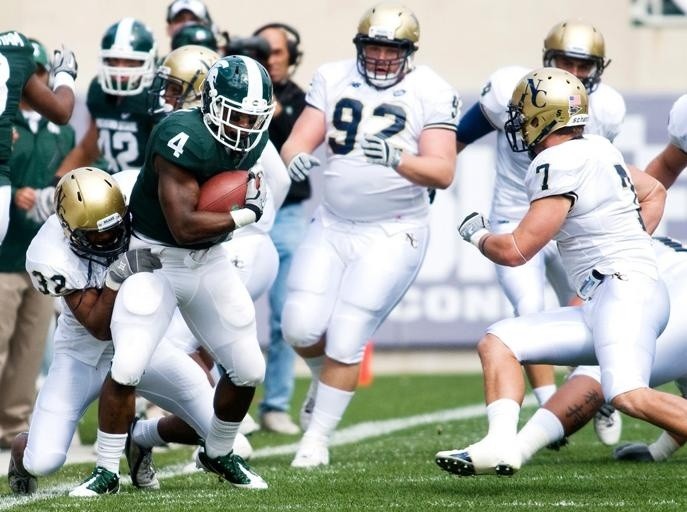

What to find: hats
[29,39,49,72]
[167,0,206,23]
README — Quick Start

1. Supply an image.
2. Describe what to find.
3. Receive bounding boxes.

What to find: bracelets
[391,147,401,169]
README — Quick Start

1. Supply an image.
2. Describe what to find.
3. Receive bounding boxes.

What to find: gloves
[34,176,61,223]
[614,442,655,462]
[54,49,78,80]
[458,213,488,242]
[245,171,265,223]
[361,135,401,168]
[288,152,320,182]
[107,248,162,283]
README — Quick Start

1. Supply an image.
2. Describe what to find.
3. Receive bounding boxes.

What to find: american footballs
[195,169,259,212]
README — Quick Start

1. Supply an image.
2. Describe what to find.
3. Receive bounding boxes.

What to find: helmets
[54,167,128,257]
[97,20,276,153]
[504,19,606,153]
[353,5,420,80]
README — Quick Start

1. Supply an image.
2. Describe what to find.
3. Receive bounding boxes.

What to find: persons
[281,3,460,469]
[0,0,313,501]
[427,17,687,479]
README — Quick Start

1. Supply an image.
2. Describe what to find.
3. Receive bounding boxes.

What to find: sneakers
[300,407,313,428]
[260,409,300,436]
[69,465,121,496]
[435,404,622,475]
[125,418,161,491]
[9,432,39,498]
[291,438,328,469]
[196,438,268,491]
